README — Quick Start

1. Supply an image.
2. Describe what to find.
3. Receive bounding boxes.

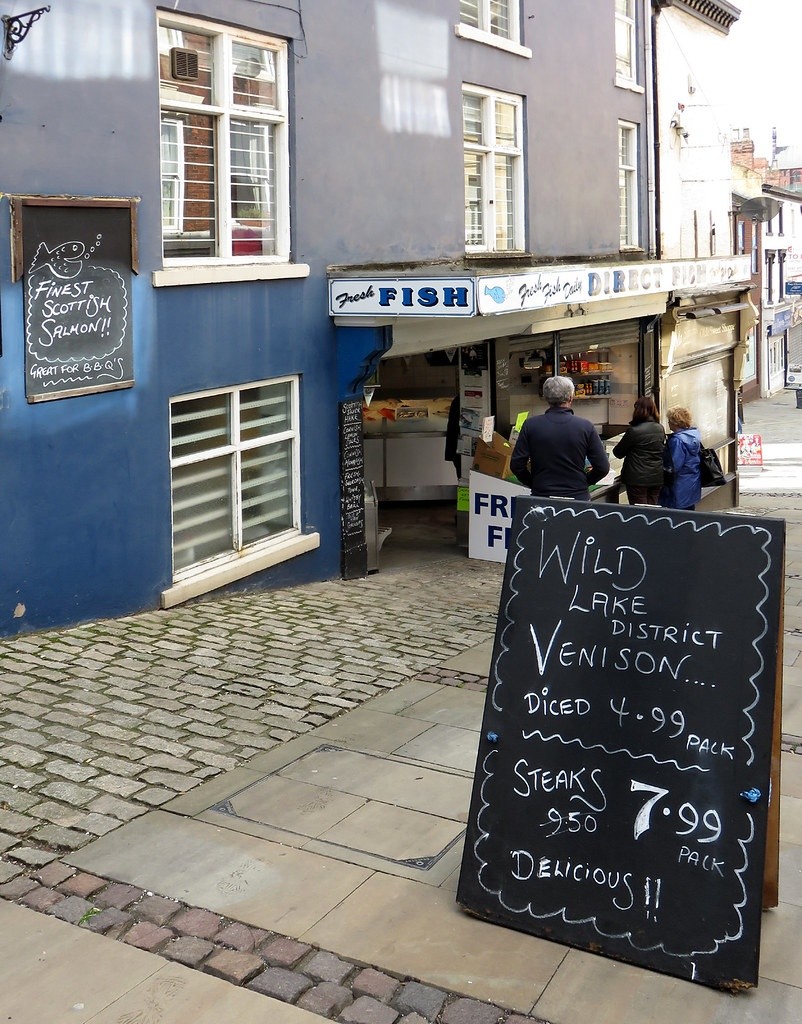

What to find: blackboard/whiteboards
[455,496,790,993]
[338,400,368,580]
[10,197,138,405]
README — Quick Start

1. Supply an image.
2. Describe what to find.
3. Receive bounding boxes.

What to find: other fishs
[484,286,505,304]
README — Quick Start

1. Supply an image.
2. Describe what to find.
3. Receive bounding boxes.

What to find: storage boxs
[472,431,514,479]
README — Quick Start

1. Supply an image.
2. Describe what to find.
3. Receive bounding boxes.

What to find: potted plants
[238,206,263,227]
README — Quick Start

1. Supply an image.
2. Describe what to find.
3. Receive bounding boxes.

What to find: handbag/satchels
[660,424,675,486]
[698,442,727,487]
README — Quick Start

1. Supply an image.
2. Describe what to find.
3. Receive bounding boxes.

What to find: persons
[613,396,666,505]
[510,376,610,501]
[667,407,702,511]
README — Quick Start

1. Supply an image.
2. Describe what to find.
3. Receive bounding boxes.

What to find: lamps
[575,303,585,315]
[524,350,542,369]
[679,302,750,319]
[564,304,574,318]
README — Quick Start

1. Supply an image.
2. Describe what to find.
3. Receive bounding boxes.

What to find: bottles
[571,361,578,371]
[585,381,592,394]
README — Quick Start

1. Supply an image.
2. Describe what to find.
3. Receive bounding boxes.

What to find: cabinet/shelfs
[540,347,613,399]
